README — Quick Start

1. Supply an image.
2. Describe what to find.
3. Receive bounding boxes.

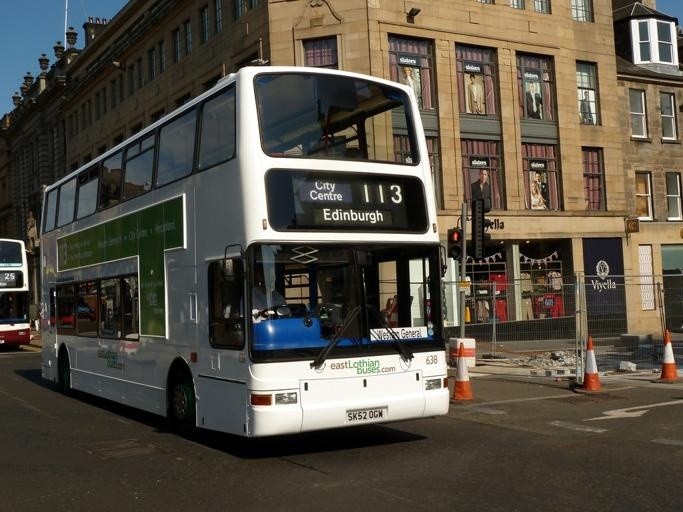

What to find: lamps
[407,7,421,18]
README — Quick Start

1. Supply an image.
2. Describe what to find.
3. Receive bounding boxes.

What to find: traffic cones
[573,334,611,395]
[446,342,481,405]
[651,329,681,386]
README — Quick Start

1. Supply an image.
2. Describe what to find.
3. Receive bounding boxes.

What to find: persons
[467,74,483,114]
[381,297,394,327]
[580,91,593,124]
[471,168,491,209]
[402,66,421,108]
[240,286,292,323]
[526,82,543,120]
[530,180,547,210]
[534,172,549,207]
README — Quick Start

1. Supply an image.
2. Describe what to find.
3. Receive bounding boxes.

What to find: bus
[45,288,75,334]
[0,238,34,350]
[38,64,454,441]
[76,303,90,322]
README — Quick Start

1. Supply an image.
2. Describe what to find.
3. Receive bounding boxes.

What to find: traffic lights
[469,196,492,261]
[444,226,463,260]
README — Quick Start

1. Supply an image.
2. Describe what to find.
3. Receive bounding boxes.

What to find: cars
[95,327,186,368]
